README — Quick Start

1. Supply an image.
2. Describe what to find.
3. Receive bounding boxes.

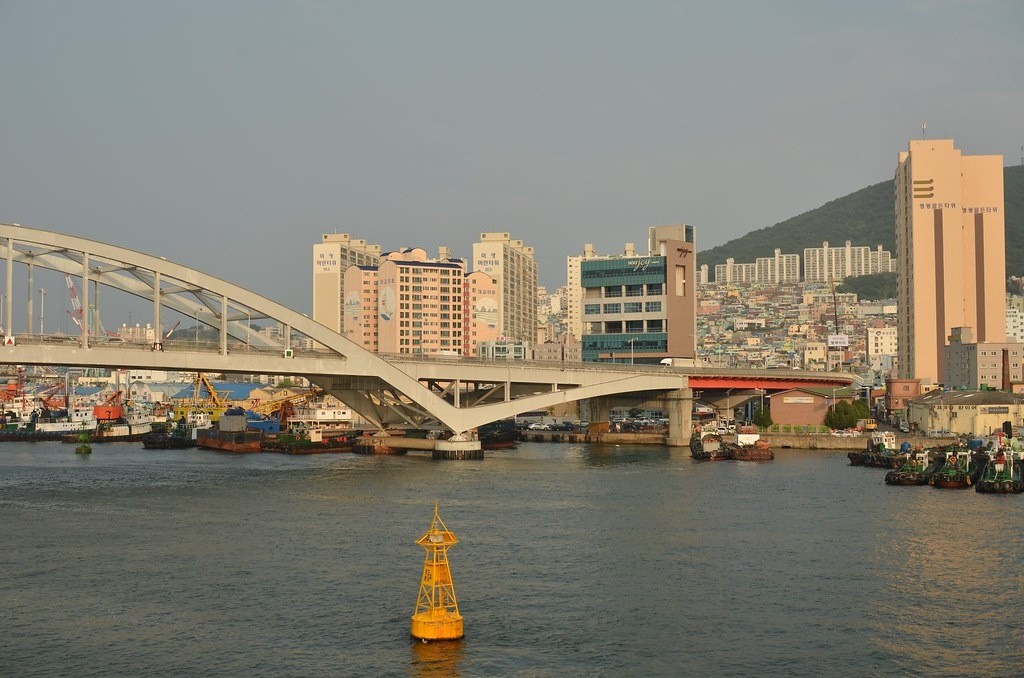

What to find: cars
[515,420,590,433]
[610,417,669,432]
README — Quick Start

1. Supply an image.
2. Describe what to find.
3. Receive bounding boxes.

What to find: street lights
[832,386,847,412]
[628,337,639,367]
[38,288,47,343]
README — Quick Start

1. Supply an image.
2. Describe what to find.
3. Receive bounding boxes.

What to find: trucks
[660,359,702,368]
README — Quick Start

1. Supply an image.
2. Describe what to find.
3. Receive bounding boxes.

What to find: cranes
[63,271,106,340]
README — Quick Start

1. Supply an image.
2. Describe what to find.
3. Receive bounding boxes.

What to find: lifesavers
[259,441,323,453]
[0,432,60,439]
[975,480,1022,491]
[950,456,957,463]
[937,472,967,483]
[864,452,904,467]
[885,471,934,482]
[234,434,245,444]
[295,435,301,441]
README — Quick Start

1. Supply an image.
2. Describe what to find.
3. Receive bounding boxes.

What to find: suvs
[930,430,955,439]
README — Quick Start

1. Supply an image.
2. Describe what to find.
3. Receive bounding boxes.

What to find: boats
[690,427,777,462]
[841,429,1024,495]
